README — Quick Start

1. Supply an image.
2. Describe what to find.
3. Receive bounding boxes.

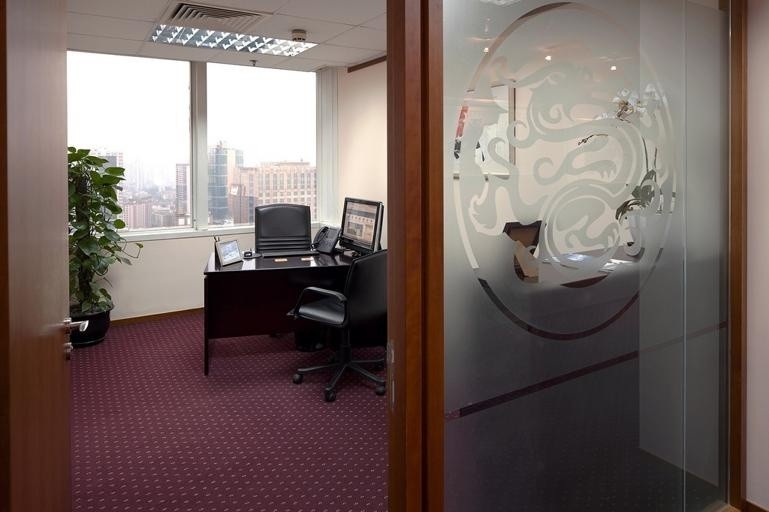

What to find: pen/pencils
[213,234,220,242]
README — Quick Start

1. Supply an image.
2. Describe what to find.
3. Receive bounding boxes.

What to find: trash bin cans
[294,330,328,352]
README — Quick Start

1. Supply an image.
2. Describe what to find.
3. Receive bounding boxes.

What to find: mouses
[244,251,252,256]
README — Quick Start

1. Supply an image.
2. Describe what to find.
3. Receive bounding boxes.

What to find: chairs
[501,221,545,270]
[252,203,312,253]
[287,249,388,404]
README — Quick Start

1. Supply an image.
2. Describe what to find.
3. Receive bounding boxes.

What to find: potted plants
[67,145,143,347]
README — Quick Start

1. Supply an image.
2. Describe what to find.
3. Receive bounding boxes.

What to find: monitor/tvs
[339,196,384,259]
[479,220,547,288]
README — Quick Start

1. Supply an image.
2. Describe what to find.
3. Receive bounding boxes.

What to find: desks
[201,246,363,376]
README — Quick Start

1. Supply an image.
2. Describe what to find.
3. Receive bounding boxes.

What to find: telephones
[314,226,341,254]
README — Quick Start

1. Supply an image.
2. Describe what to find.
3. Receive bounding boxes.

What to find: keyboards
[262,251,319,258]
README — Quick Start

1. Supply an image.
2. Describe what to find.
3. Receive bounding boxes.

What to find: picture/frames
[214,238,243,266]
[453,78,517,181]
